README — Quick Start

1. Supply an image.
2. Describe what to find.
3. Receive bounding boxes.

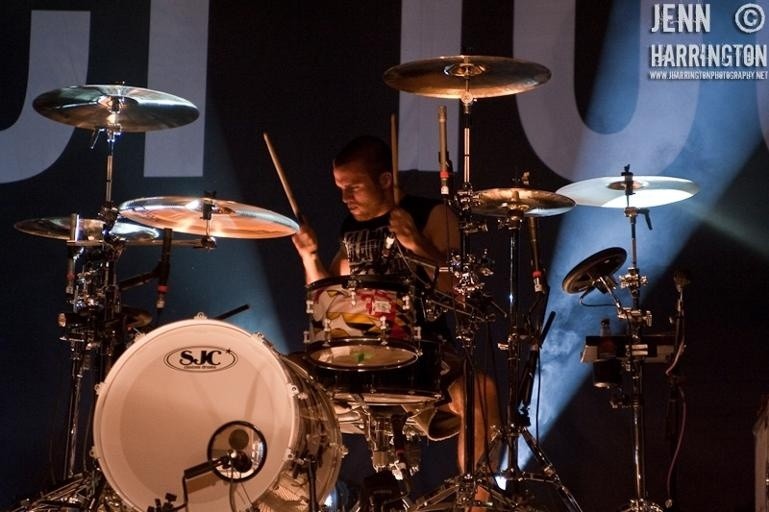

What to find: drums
[89,313,346,512]
[303,275,426,370]
[305,291,445,404]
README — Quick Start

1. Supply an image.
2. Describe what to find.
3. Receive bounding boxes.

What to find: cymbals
[556,175,698,209]
[118,196,300,238]
[461,189,575,218]
[14,218,160,245]
[382,55,551,100]
[32,85,200,132]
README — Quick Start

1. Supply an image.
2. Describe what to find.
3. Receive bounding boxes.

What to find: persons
[289,127,507,511]
